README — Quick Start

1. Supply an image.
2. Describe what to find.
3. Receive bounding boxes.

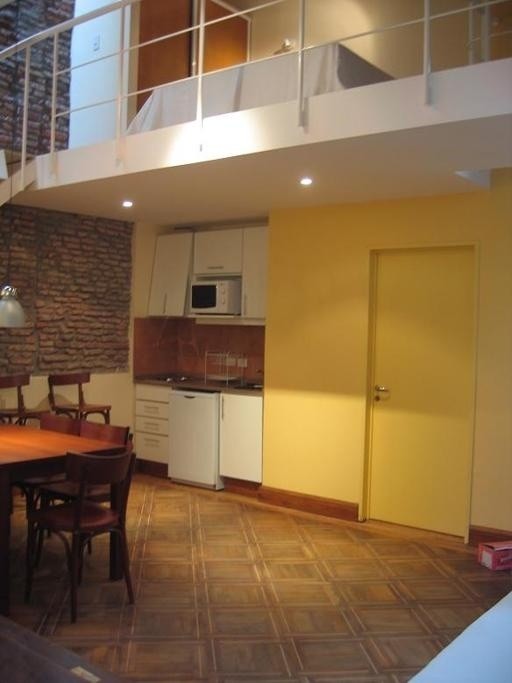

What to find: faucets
[255,368,263,374]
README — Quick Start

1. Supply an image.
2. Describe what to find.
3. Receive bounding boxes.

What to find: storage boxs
[476,541,512,571]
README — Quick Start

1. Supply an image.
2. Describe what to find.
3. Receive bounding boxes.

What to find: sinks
[233,382,263,391]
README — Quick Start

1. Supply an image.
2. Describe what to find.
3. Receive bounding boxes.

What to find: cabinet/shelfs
[218,394,262,486]
[192,228,243,278]
[135,381,170,466]
[147,231,193,317]
[241,225,268,320]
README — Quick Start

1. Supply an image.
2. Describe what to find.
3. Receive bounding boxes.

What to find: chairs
[0,370,138,625]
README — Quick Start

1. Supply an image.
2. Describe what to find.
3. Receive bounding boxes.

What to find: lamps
[0,0,27,329]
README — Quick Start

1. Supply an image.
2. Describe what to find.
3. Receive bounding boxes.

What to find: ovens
[168,387,224,492]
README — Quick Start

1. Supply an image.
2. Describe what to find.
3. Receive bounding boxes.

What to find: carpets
[0,614,128,683]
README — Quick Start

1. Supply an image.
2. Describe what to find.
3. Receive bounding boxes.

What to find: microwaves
[190,279,240,316]
